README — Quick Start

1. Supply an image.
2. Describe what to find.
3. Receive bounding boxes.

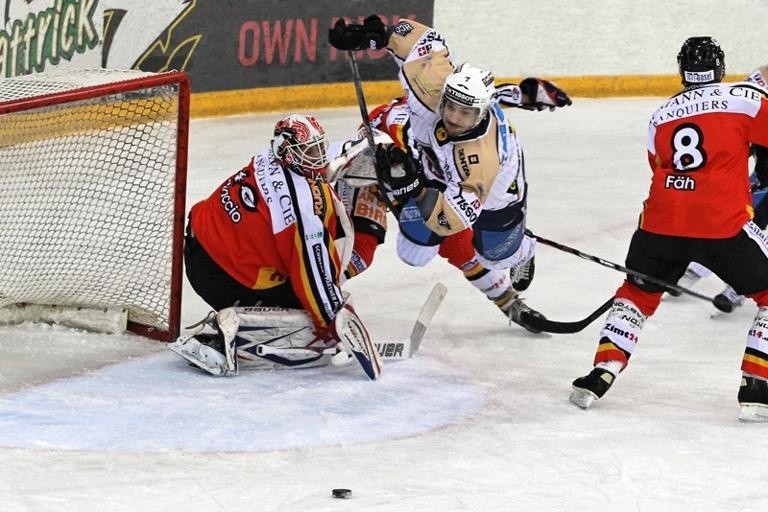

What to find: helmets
[678,35,725,87]
[267,114,330,180]
[436,60,496,138]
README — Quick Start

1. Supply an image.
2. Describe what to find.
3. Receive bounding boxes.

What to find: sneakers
[177,328,233,368]
[710,285,746,313]
[737,375,768,406]
[570,365,616,398]
[500,298,548,333]
[510,229,537,292]
[667,268,701,298]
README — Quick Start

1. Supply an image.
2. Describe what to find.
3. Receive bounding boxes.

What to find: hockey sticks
[257,282,448,361]
[520,295,617,332]
[524,231,744,305]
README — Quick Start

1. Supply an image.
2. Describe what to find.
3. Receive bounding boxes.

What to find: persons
[327,13,535,292]
[183,113,355,375]
[665,65,768,313]
[337,79,573,283]
[571,36,768,404]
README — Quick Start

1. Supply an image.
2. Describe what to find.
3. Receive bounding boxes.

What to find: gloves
[519,76,568,112]
[374,141,426,202]
[329,13,391,54]
